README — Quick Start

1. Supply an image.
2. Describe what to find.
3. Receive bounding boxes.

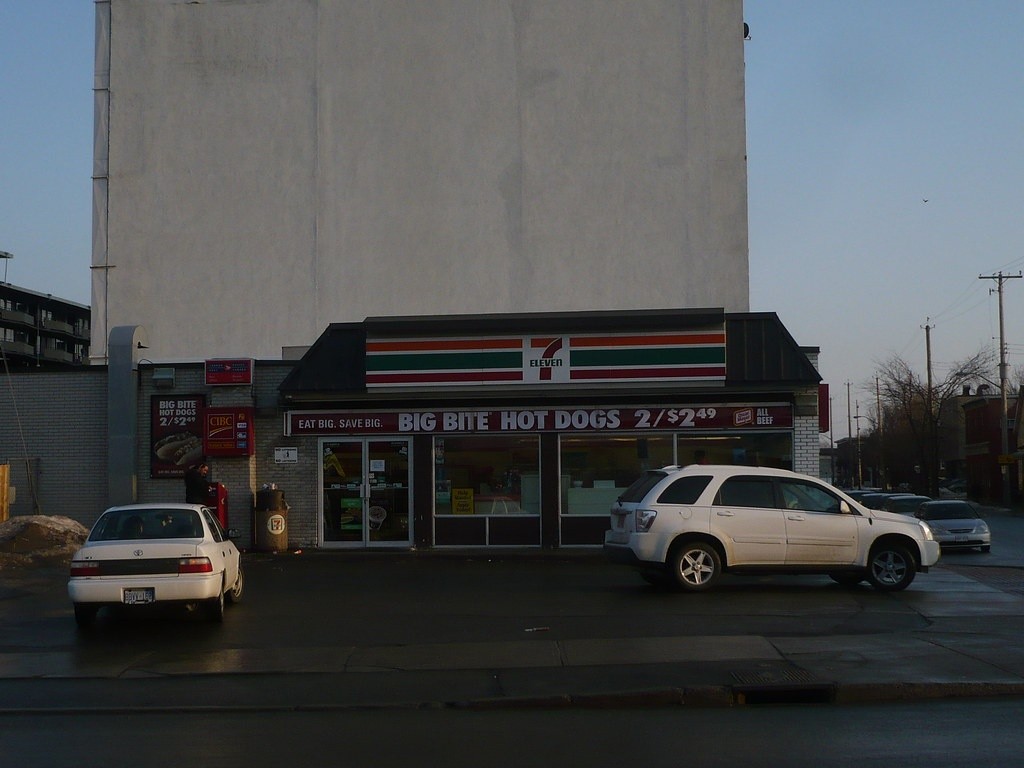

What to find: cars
[67,503,244,627]
[843,489,933,517]
[914,499,992,552]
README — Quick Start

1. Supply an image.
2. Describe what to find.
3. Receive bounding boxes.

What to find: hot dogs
[172,438,202,465]
[153,431,197,459]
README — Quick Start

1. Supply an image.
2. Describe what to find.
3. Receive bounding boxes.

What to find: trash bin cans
[255,489,288,552]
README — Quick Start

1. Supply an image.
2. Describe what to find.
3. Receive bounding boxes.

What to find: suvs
[604,462,940,591]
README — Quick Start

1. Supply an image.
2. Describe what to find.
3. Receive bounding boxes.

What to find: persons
[183,463,217,506]
